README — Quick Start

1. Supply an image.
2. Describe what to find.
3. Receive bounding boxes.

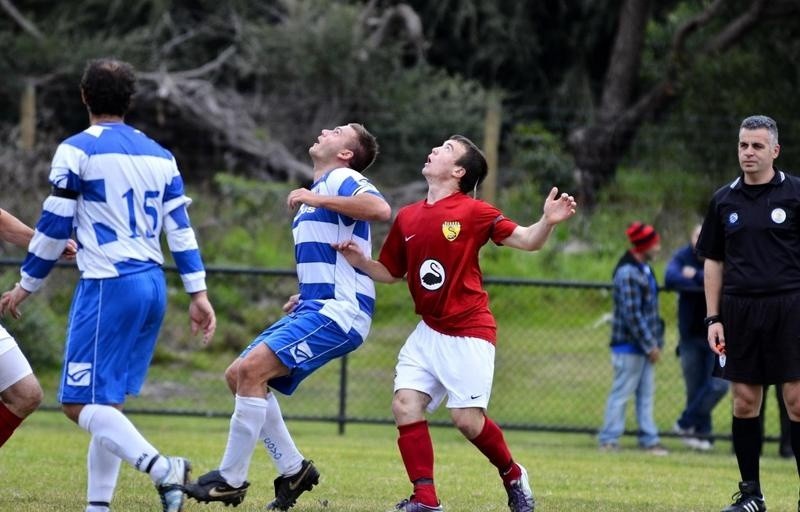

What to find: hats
[626,222,660,250]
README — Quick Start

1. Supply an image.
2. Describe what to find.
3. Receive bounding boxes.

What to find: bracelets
[704,312,723,326]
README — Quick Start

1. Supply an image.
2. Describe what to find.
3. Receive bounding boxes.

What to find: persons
[594,219,672,456]
[329,133,581,511]
[179,119,392,510]
[664,219,730,453]
[699,112,799,511]
[0,53,218,510]
[0,208,79,454]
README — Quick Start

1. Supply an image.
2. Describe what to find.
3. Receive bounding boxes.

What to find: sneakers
[156,453,320,512]
[721,481,766,511]
[389,495,444,511]
[502,464,535,511]
